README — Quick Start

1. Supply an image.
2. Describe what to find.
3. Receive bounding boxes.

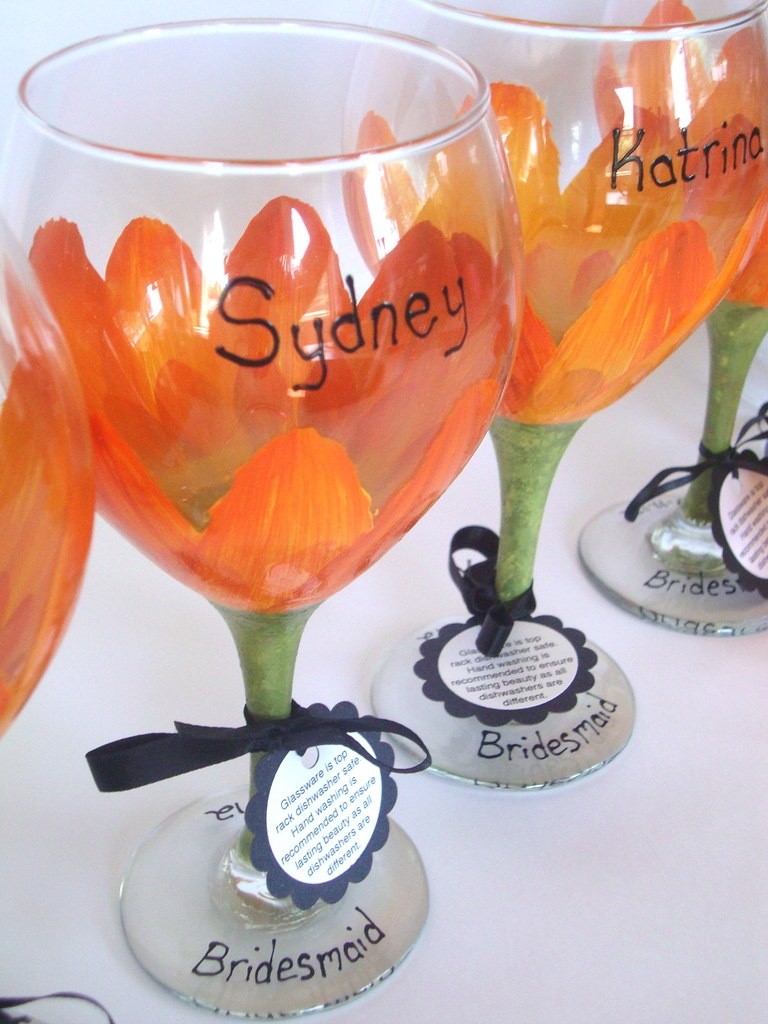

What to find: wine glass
[342,3,768,788]
[578,2,766,635]
[6,18,525,1017]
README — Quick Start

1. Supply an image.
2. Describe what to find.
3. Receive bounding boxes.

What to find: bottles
[1,224,110,1023]
